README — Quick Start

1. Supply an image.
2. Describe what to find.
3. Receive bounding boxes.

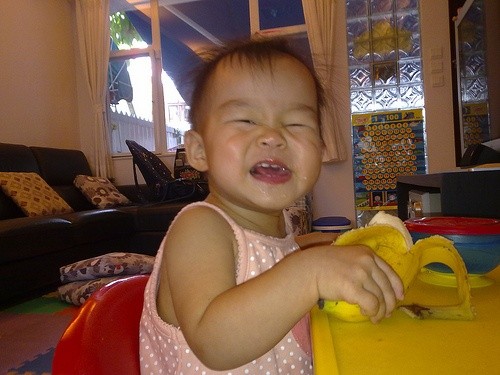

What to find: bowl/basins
[404,216,500,275]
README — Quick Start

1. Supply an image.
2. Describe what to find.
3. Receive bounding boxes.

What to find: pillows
[73,175,131,210]
[58,251,155,306]
[0,172,73,217]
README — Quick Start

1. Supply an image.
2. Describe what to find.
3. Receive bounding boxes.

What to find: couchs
[0,143,186,311]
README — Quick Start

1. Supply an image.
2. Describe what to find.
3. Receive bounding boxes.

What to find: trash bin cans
[311,217,352,233]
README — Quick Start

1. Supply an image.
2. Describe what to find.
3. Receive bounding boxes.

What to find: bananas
[319,209,473,321]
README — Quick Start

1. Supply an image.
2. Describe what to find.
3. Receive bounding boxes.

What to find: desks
[310,267,500,375]
[397,169,500,221]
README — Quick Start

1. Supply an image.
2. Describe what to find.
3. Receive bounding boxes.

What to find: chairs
[126,140,208,205]
[50,274,149,375]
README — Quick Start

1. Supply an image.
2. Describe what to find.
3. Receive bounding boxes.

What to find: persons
[138,38,406,375]
